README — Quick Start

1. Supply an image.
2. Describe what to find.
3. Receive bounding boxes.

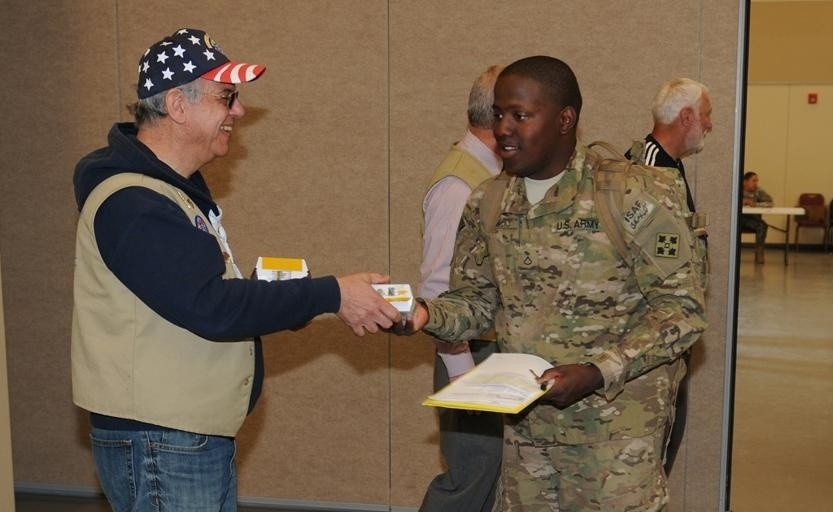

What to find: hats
[136,27,267,97]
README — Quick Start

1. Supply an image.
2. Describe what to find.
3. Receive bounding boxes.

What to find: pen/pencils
[540,382,547,390]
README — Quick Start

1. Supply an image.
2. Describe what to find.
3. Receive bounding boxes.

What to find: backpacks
[480,140,711,295]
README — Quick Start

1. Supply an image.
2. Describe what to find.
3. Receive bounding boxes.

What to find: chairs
[794,193,827,253]
[828,199,833,252]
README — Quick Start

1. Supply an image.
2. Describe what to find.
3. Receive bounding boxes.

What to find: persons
[377,55,712,512]
[419,65,508,512]
[624,78,711,478]
[740,172,774,264]
[69,28,404,512]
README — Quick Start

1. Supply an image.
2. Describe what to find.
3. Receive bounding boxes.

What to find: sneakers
[756,247,765,266]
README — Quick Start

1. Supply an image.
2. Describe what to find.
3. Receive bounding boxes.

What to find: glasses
[183,87,240,109]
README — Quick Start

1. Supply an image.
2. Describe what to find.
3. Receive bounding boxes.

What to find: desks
[741,205,807,267]
